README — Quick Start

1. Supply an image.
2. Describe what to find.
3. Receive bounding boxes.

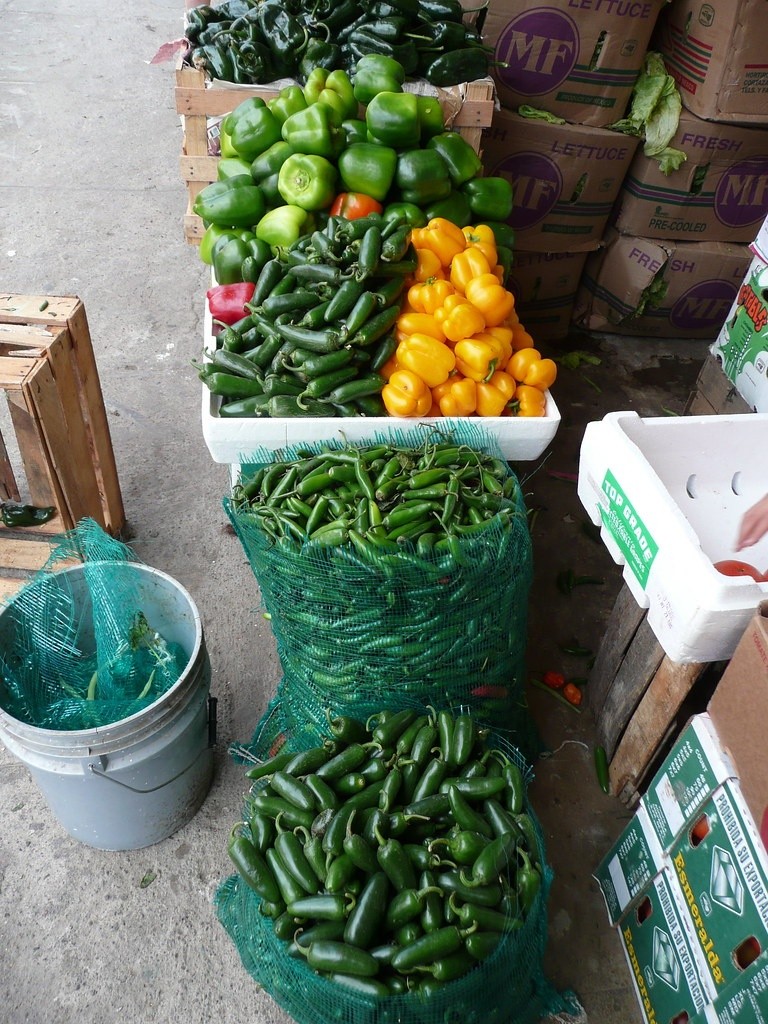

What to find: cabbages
[517,50,687,367]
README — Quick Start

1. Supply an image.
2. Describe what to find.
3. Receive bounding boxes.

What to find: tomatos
[714,560,768,582]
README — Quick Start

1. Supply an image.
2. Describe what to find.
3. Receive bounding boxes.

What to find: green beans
[59,668,154,728]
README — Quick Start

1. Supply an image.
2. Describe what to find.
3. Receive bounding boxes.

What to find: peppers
[226,420,608,1024]
[183,0,510,86]
[0,503,56,527]
[193,51,557,420]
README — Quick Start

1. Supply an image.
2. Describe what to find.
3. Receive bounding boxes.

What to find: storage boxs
[583,576,704,806]
[202,292,561,464]
[706,598,768,854]
[458,0,768,337]
[577,410,768,664]
[176,36,495,247]
[709,213,768,412]
[0,293,126,607]
[588,713,768,1024]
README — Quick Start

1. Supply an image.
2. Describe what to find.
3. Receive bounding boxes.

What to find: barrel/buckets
[1,561,218,850]
